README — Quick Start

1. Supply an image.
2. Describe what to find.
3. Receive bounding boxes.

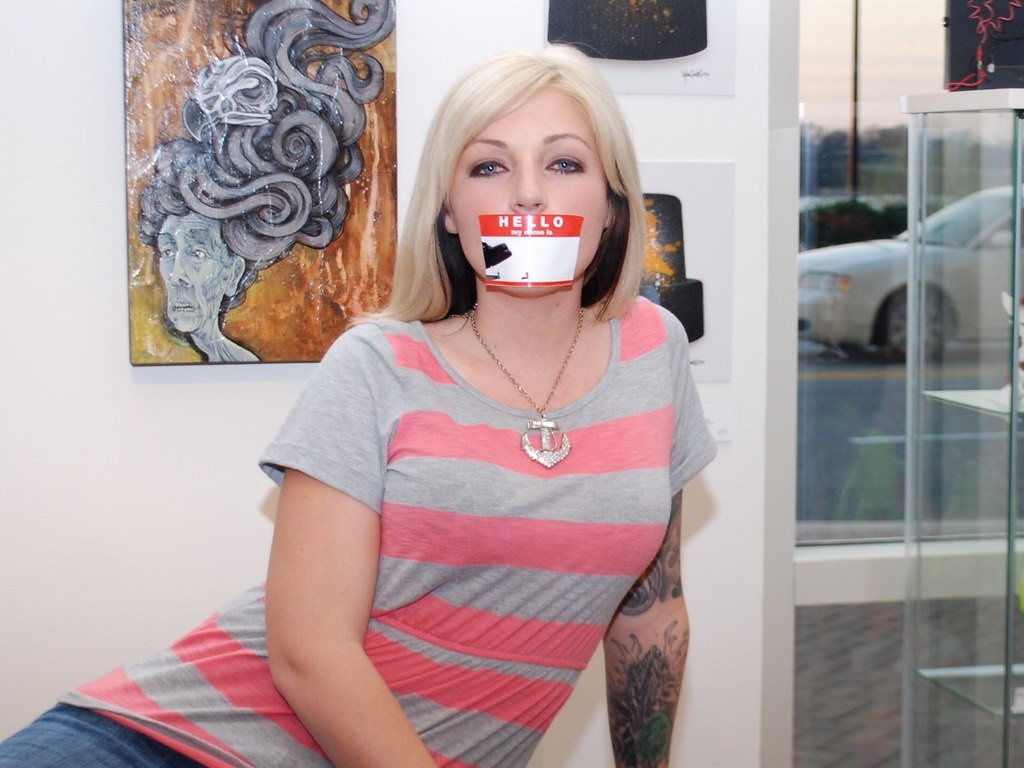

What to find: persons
[0,48,717,768]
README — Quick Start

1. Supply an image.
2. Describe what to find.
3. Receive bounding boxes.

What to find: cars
[797,186,1024,364]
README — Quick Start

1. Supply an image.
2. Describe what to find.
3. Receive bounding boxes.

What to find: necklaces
[470,302,584,466]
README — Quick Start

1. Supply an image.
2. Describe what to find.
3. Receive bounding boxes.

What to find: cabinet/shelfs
[895,89,1024,768]
